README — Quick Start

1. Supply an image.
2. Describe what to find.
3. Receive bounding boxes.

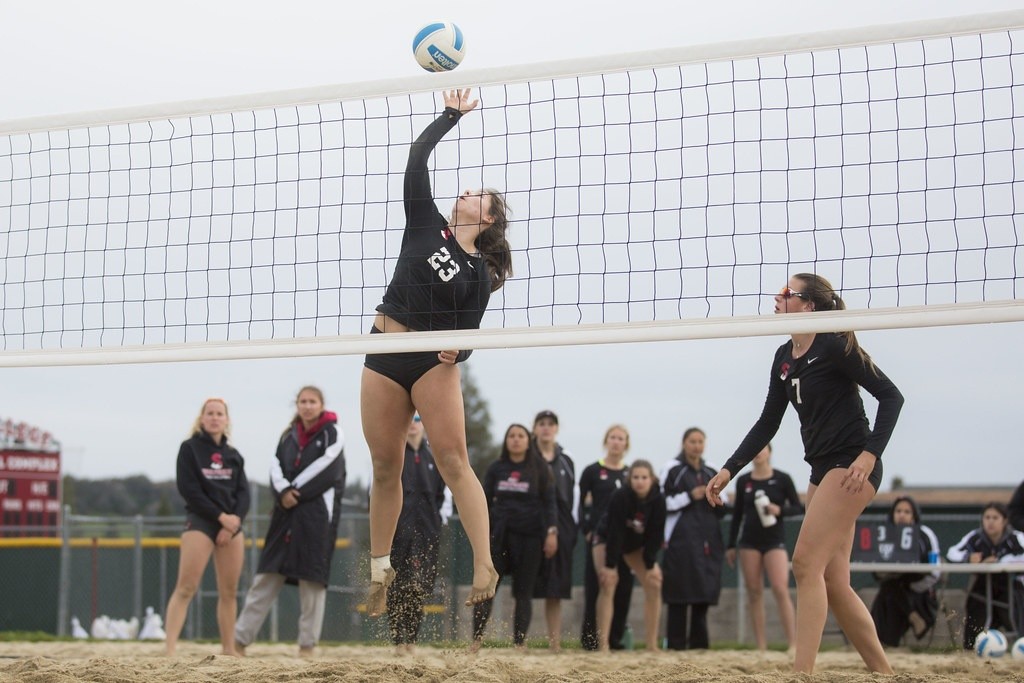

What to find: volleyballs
[412,18,466,72]
[974,628,1008,658]
[1011,636,1024,663]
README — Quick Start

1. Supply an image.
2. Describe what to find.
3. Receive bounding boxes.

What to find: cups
[928,551,938,564]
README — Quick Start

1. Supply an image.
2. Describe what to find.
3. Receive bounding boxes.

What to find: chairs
[925,556,958,649]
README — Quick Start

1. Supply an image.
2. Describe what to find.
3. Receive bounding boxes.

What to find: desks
[851,560,1024,653]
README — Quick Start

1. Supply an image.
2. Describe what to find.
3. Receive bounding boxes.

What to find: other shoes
[915,621,929,639]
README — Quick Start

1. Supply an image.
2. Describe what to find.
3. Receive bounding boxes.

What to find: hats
[536,411,558,424]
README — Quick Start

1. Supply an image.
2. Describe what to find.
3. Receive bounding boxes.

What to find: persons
[466,425,558,653]
[385,412,453,656]
[233,388,346,656]
[665,428,722,650]
[706,273,906,674]
[945,504,1024,648]
[727,444,802,654]
[529,410,577,653]
[360,86,512,613]
[163,399,250,657]
[592,460,667,651]
[872,496,942,647]
[577,424,634,651]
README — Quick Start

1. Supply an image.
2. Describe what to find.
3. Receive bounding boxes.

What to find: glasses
[412,416,421,423]
[781,288,809,301]
